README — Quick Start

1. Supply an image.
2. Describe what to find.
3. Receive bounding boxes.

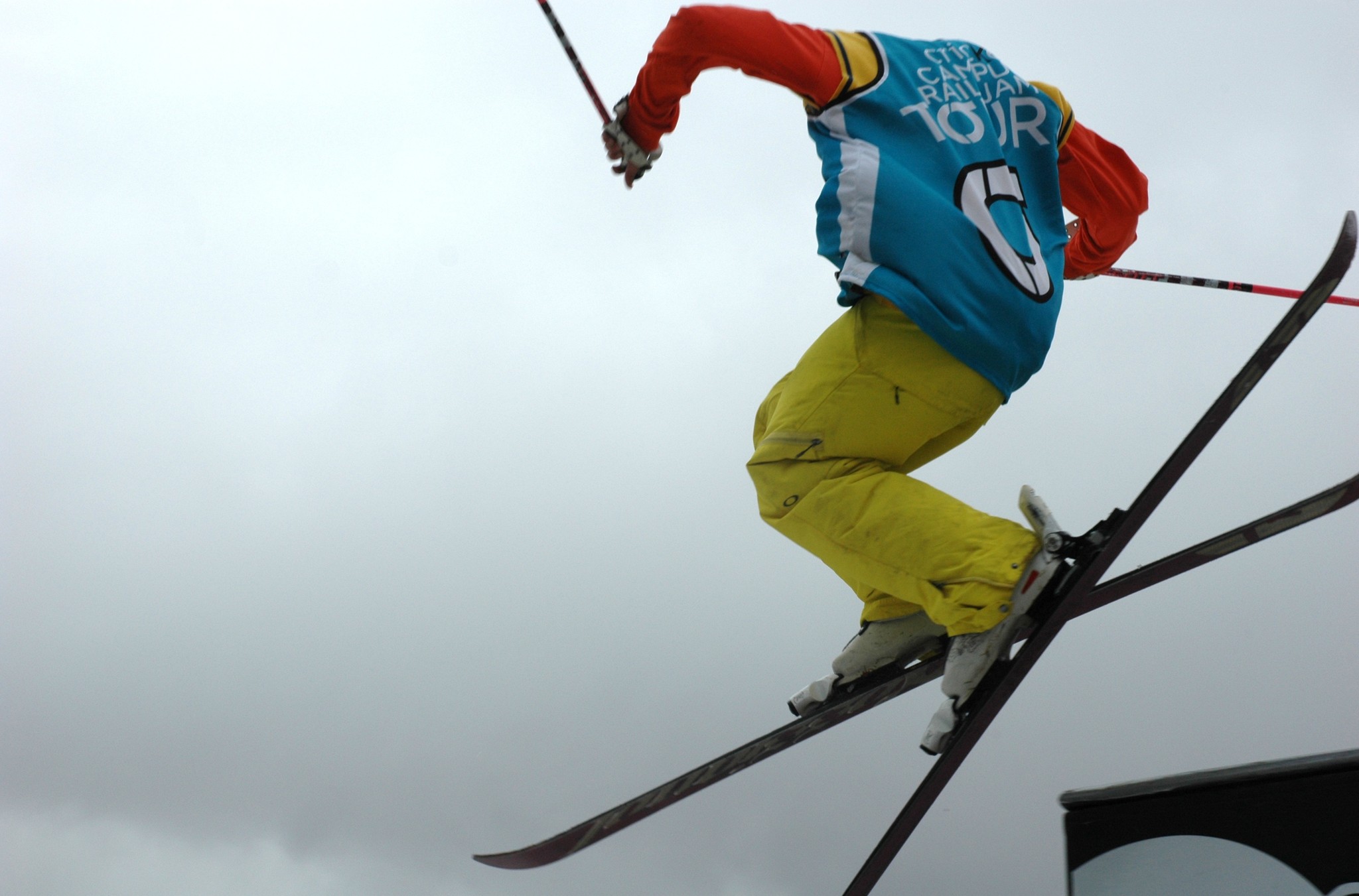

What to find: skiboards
[469,209,1359,896]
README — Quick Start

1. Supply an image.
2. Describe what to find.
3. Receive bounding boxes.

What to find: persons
[601,5,1149,752]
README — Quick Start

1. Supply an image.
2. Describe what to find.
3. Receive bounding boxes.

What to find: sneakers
[832,608,946,677]
[941,546,1047,698]
